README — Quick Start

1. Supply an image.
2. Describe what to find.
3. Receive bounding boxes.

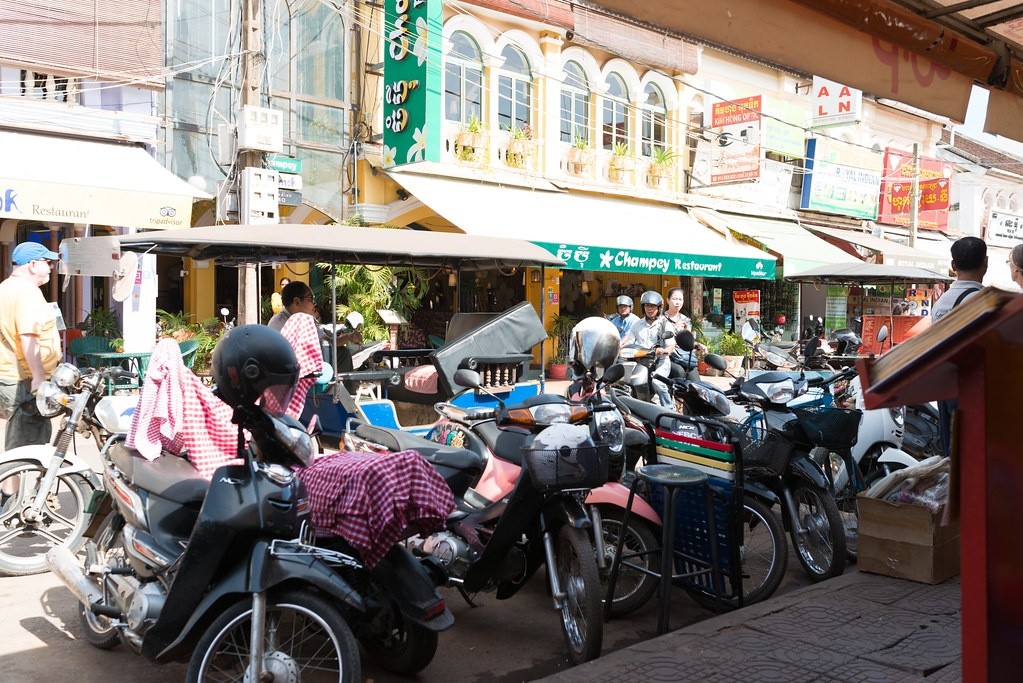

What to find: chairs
[642,412,745,610]
[58,330,201,386]
[427,334,445,349]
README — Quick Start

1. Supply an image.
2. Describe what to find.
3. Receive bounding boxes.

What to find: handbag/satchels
[670,346,698,367]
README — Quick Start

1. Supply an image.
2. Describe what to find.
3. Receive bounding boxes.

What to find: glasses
[27,258,52,267]
[300,294,315,304]
[1006,259,1012,266]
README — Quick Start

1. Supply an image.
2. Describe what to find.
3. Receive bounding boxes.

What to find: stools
[604,464,724,635]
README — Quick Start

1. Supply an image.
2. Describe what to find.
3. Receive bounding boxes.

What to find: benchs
[305,370,395,406]
[374,312,534,405]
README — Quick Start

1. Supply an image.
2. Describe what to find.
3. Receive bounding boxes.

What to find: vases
[508,142,526,154]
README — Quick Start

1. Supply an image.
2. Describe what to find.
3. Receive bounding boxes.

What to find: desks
[85,353,152,396]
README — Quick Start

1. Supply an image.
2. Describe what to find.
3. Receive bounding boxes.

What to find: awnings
[388,173,778,279]
[1,128,213,229]
[689,207,954,279]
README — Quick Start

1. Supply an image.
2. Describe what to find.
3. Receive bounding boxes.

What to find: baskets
[520,445,611,510]
[793,405,862,446]
[726,420,793,480]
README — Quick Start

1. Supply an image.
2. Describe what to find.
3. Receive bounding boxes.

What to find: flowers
[508,124,534,142]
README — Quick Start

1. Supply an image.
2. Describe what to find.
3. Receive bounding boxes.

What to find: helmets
[346,311,364,330]
[837,331,863,354]
[568,317,620,377]
[529,423,599,484]
[616,295,633,313]
[640,291,663,316]
[211,326,299,413]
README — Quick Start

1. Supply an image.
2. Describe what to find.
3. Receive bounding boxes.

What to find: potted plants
[648,146,683,187]
[547,312,578,375]
[609,141,629,182]
[570,127,592,163]
[690,312,756,376]
[455,113,482,149]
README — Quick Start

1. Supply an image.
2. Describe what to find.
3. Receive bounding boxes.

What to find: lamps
[663,280,670,288]
[531,269,540,283]
[180,270,188,277]
[448,274,457,286]
[582,281,591,296]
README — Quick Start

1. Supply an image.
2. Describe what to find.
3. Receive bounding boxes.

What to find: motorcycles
[1,304,945,683]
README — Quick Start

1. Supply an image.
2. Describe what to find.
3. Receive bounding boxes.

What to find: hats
[12,241,60,265]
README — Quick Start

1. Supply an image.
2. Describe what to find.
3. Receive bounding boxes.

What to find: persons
[1005,244,1023,289]
[893,302,911,316]
[661,288,708,382]
[269,278,364,348]
[612,296,640,339]
[931,236,988,457]
[0,241,63,502]
[620,291,676,409]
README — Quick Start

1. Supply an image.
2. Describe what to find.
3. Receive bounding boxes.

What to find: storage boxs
[856,490,960,585]
